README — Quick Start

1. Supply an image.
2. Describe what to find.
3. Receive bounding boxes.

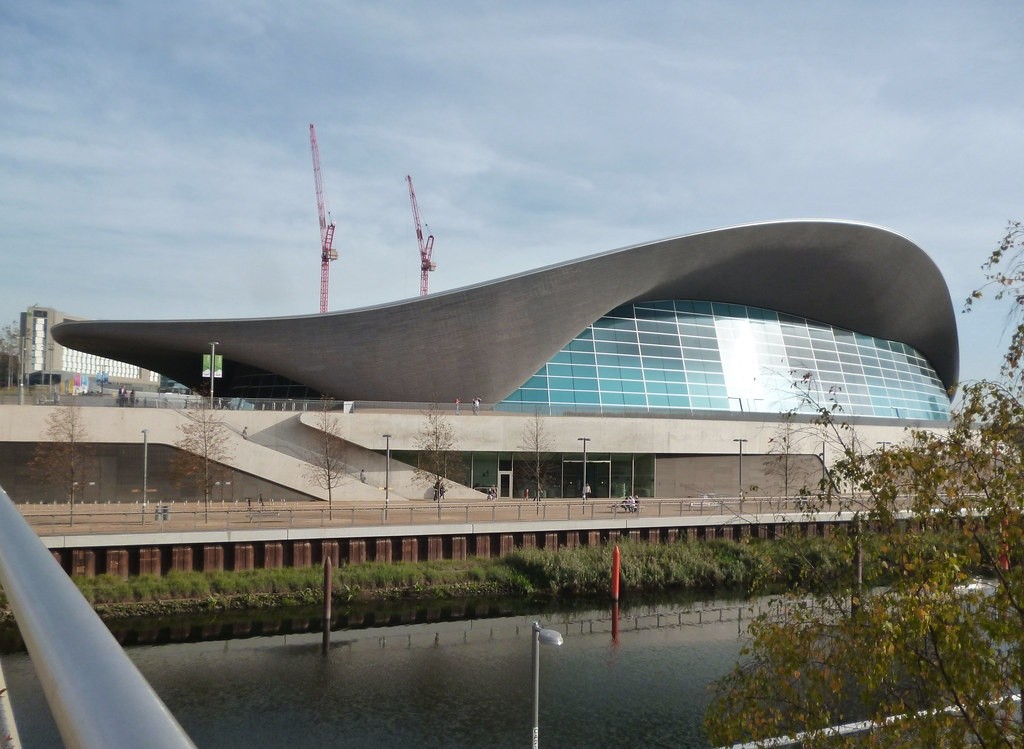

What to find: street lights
[876,441,891,452]
[208,342,219,410]
[383,435,391,520]
[578,438,591,515]
[18,337,32,405]
[531,622,565,749]
[142,429,149,527]
[733,439,747,493]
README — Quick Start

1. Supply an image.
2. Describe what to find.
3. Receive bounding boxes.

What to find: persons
[487,485,497,501]
[242,427,248,440]
[455,397,461,416]
[586,483,591,500]
[360,469,365,483]
[522,488,528,501]
[472,396,481,415]
[621,495,640,513]
[118,385,135,407]
[533,485,543,501]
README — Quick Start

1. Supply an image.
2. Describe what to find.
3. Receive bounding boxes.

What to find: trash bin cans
[53,392,60,405]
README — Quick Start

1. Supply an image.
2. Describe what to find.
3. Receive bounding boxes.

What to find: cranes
[405,175,437,297]
[310,124,339,314]
